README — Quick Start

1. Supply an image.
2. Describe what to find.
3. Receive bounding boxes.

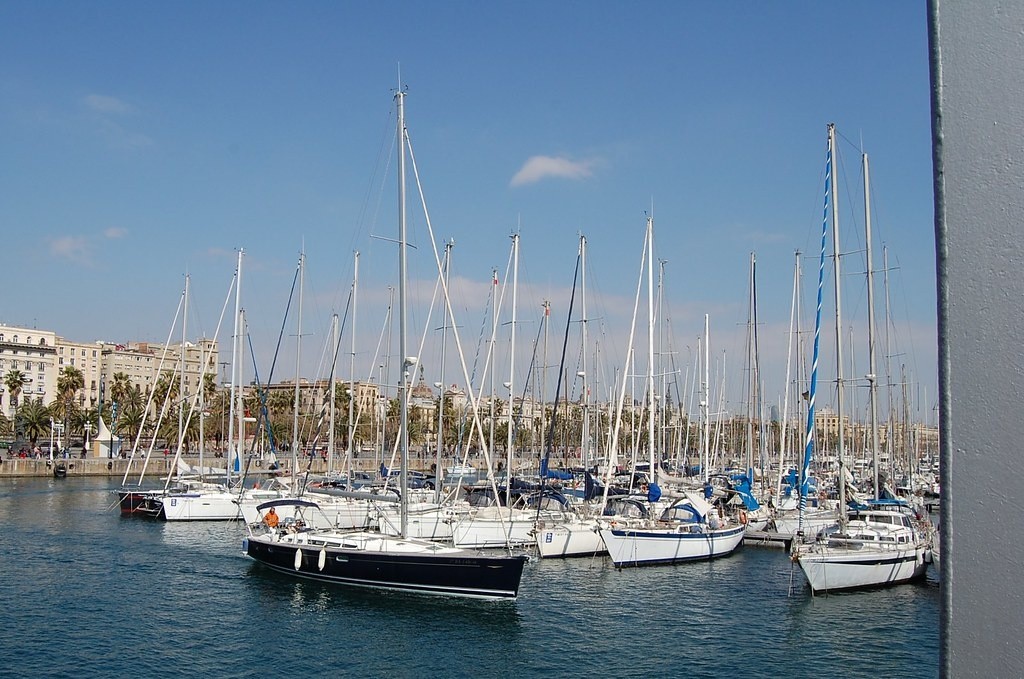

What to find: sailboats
[109,59,939,605]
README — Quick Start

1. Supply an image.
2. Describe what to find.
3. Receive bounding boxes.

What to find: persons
[261,507,279,535]
[140,447,146,460]
[353,450,358,458]
[163,448,168,459]
[118,447,127,459]
[6,445,88,459]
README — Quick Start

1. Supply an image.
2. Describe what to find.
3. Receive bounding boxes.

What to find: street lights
[50,417,54,460]
[84,420,92,453]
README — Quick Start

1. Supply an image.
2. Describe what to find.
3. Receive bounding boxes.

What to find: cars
[37,441,59,455]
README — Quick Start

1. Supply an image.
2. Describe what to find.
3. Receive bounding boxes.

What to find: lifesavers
[54,464,67,476]
